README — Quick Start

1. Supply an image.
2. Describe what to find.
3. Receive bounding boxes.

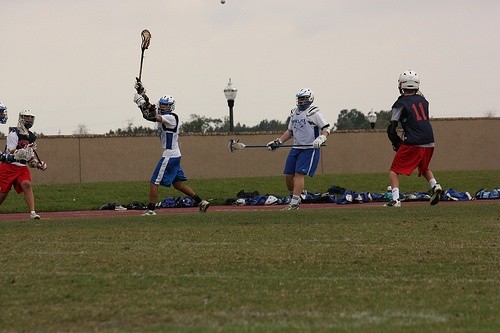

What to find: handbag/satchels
[336,188,500,205]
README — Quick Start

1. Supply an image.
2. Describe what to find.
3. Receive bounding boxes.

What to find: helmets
[295,88,314,110]
[19,108,35,128]
[398,71,420,90]
[0,103,8,124]
[157,96,175,113]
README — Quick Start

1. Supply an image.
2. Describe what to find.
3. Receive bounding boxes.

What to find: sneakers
[141,210,156,216]
[384,199,401,207]
[429,183,442,205]
[198,200,209,212]
[30,213,40,219]
[288,195,301,210]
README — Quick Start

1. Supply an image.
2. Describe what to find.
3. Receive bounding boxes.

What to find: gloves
[135,77,146,94]
[37,160,47,171]
[266,138,282,151]
[313,135,326,149]
[24,142,37,151]
[134,93,146,106]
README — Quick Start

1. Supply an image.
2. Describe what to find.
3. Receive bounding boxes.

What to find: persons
[266,88,330,211]
[133,77,210,216]
[0,105,48,220]
[384,70,442,207]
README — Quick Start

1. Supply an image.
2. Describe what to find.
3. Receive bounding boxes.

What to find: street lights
[222,77,238,132]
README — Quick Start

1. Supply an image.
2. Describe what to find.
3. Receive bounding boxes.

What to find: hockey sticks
[135,28,152,97]
[16,116,43,165]
[228,137,328,152]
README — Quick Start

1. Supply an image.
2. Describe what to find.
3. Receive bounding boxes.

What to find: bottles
[387,185,392,192]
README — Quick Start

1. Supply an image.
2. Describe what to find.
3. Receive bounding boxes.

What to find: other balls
[221,0,225,4]
